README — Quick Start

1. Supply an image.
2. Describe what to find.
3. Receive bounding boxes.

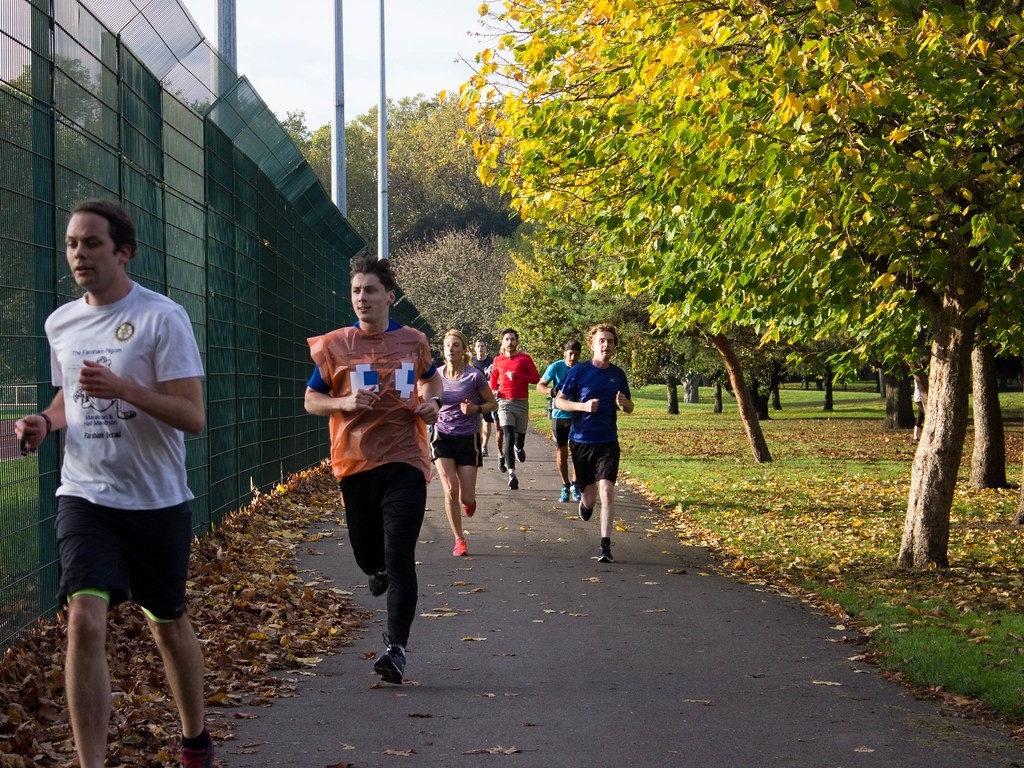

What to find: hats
[565,339,581,352]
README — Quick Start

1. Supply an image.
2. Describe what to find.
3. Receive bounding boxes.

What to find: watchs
[477,404,483,416]
[428,396,442,408]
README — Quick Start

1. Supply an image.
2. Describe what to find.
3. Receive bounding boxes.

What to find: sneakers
[498,454,507,472]
[181,739,214,768]
[514,444,527,463]
[579,498,593,521]
[368,570,389,596]
[559,487,570,502]
[464,500,476,518]
[373,632,406,685]
[570,485,582,502]
[453,539,468,556]
[597,542,616,563]
[482,447,488,457]
[508,476,518,490]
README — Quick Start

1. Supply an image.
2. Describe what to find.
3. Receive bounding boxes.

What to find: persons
[14,198,215,768]
[488,345,507,474]
[490,326,540,491]
[304,250,445,685]
[536,338,583,502]
[469,339,495,457]
[555,323,634,563]
[417,328,499,557]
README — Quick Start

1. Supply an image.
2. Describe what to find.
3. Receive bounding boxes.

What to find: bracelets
[38,412,52,435]
